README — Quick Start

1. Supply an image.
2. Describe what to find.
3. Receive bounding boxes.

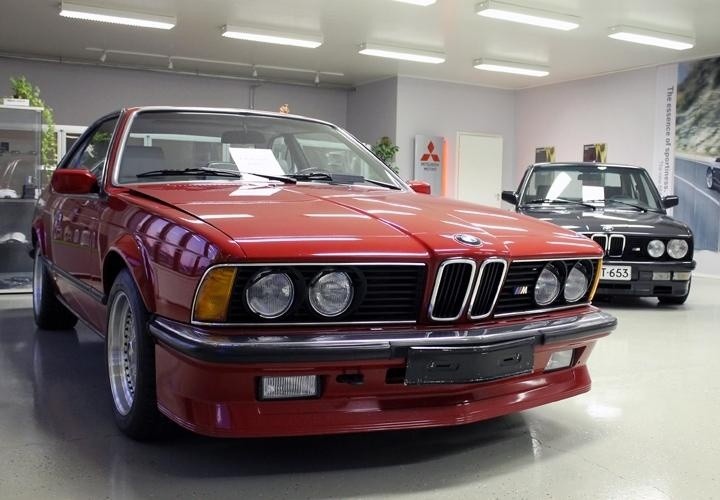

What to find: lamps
[474,59,548,79]
[58,3,175,31]
[220,24,322,50]
[607,25,694,51]
[357,44,446,65]
[476,0,580,32]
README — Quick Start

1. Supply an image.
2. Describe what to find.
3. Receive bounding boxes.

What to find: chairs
[118,145,176,177]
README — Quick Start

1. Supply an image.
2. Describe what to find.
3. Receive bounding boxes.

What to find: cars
[705,155,720,192]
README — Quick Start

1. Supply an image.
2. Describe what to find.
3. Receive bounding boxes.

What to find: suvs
[501,157,694,309]
[26,104,619,445]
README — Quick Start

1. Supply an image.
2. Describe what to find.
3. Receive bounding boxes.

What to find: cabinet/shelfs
[1,105,44,295]
